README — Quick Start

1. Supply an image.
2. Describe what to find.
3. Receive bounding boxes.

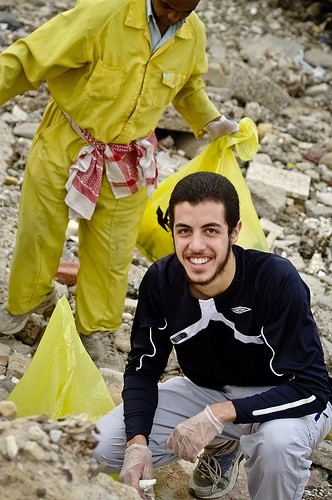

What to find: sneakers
[1,283,68,335]
[188,432,246,500]
[79,334,102,361]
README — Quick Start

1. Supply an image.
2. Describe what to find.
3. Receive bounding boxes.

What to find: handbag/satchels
[7,298,117,426]
[133,118,270,270]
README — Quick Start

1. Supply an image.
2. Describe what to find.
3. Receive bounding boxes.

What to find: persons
[93,171,332,500]
[1,1,268,374]
